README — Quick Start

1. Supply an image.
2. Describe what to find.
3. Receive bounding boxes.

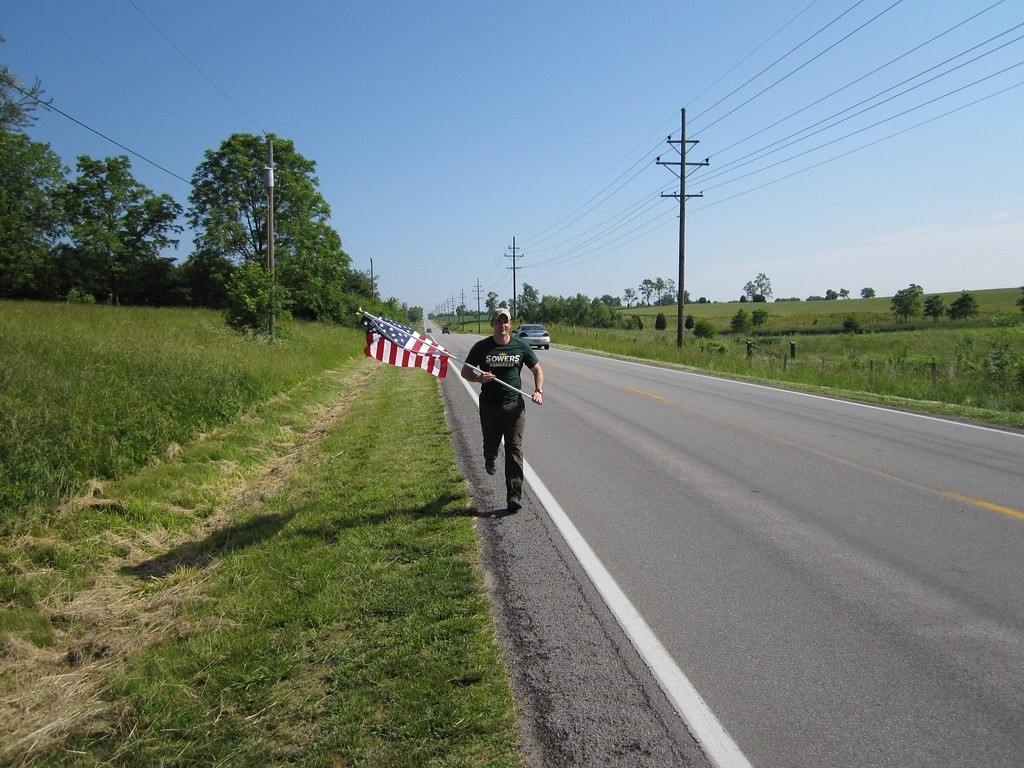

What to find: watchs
[535,388,543,394]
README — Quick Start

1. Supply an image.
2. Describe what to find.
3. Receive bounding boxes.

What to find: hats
[490,308,511,321]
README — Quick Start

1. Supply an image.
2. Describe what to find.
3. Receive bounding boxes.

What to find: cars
[511,324,551,349]
[427,328,432,333]
[442,328,450,334]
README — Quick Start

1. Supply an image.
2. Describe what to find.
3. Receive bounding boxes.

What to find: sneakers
[485,459,497,475]
[507,494,522,513]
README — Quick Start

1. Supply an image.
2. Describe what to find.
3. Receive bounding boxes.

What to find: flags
[360,313,451,378]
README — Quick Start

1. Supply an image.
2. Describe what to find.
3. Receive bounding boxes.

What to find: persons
[461,308,544,512]
